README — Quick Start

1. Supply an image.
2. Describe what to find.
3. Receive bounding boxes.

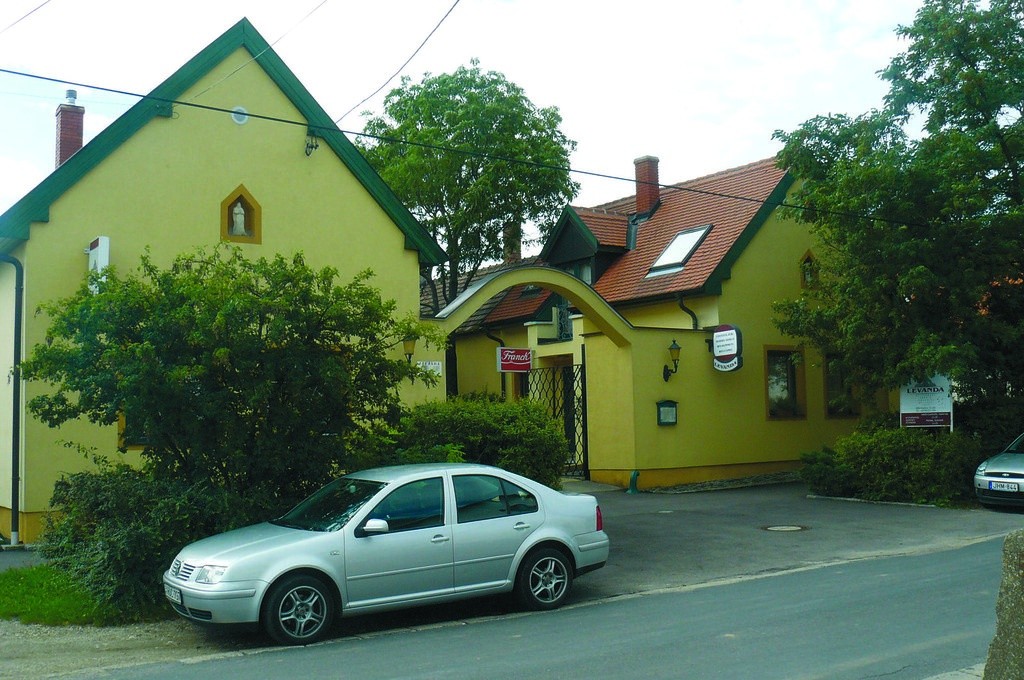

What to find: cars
[163,458,609,647]
[971,433,1024,514]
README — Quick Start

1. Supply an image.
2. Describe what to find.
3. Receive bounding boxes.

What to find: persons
[233,202,245,233]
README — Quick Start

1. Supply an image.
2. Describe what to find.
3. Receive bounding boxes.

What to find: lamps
[661,341,682,382]
[403,332,419,363]
